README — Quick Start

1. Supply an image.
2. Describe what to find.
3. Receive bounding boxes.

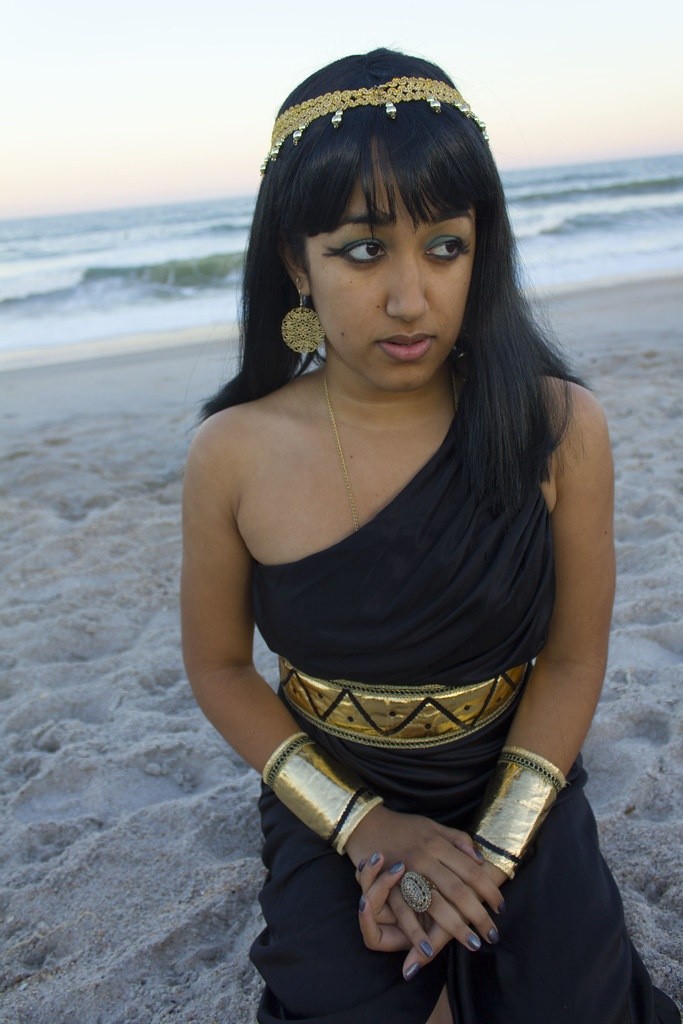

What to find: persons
[181,51,682,1024]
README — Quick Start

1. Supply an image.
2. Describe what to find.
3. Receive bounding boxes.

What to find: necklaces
[321,369,461,533]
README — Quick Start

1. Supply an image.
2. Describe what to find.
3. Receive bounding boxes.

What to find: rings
[399,870,435,912]
